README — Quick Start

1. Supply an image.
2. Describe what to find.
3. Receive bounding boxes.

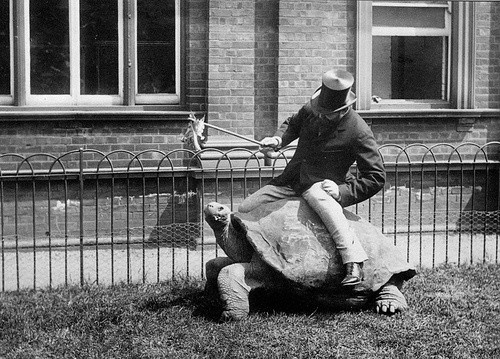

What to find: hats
[310,68,357,115]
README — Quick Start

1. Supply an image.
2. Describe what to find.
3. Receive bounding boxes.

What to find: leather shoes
[341,263,367,286]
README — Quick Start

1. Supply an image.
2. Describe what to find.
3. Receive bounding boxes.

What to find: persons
[237,68,386,288]
